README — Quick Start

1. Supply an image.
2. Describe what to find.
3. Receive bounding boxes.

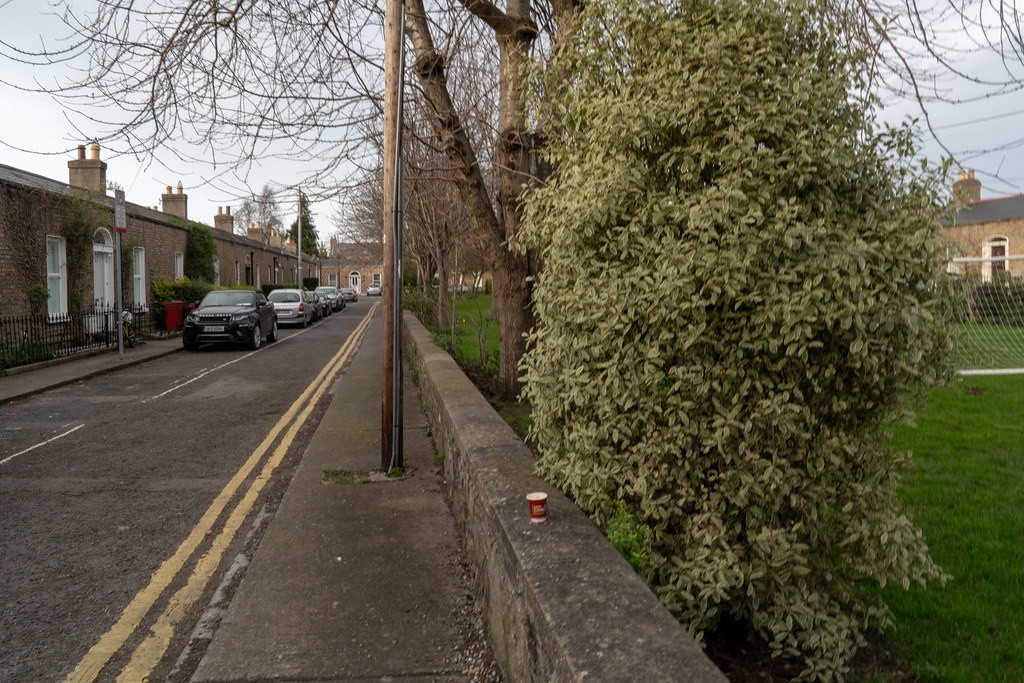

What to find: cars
[302,285,346,321]
[266,288,313,328]
[341,287,358,303]
[182,289,279,351]
[366,283,381,297]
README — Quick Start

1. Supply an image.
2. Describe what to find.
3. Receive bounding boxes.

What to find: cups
[526,492,547,522]
